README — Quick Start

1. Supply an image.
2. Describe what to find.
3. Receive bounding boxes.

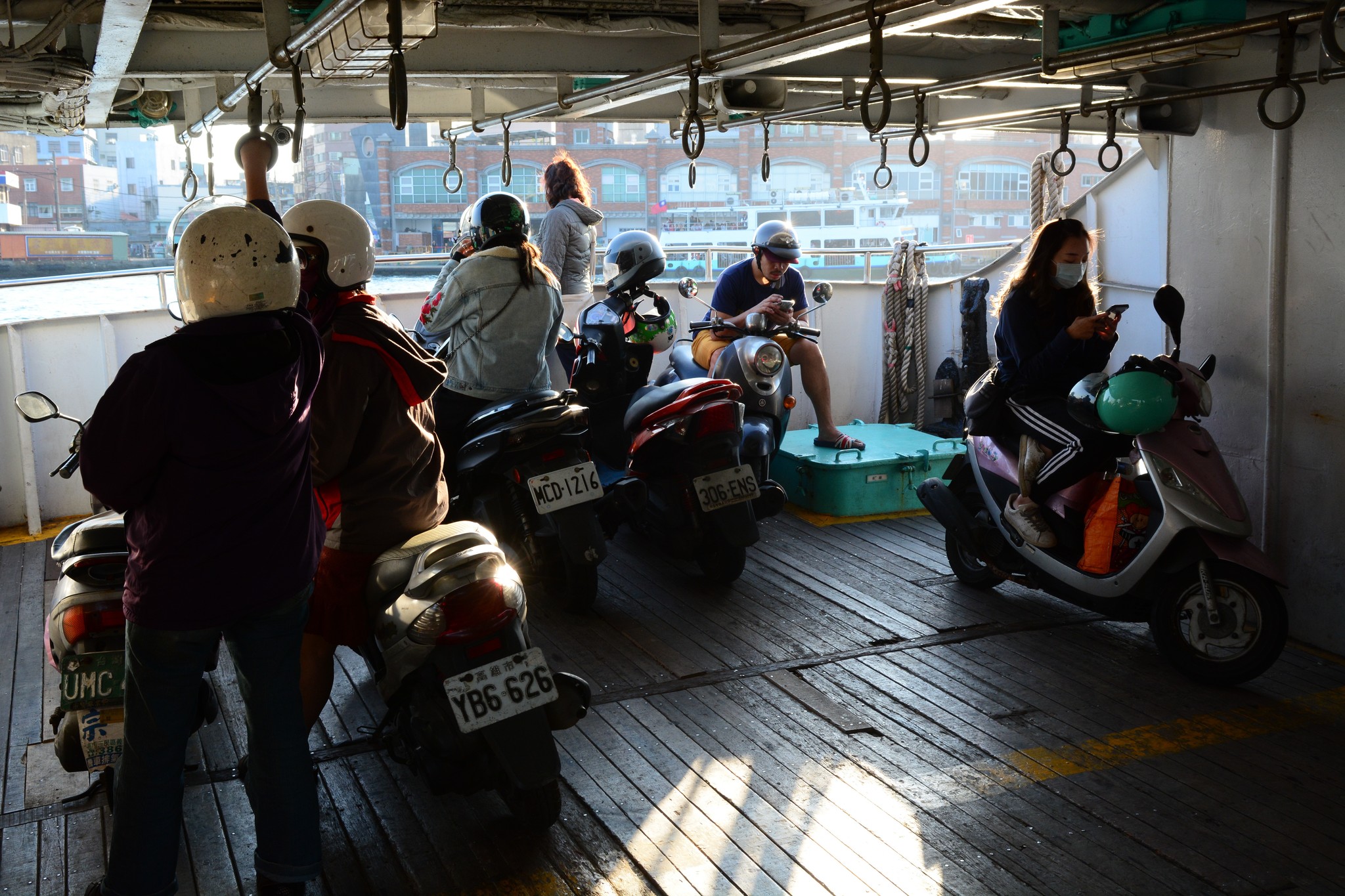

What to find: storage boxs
[769,419,968,518]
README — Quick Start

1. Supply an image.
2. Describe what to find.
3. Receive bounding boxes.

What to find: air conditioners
[725,194,739,207]
[768,191,782,205]
[795,188,808,194]
[839,191,854,203]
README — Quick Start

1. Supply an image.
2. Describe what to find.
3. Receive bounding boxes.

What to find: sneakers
[1004,494,1059,548]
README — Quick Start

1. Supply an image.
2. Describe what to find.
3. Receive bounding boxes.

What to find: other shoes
[86,876,104,896]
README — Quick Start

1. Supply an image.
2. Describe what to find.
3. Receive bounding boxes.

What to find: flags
[651,199,668,215]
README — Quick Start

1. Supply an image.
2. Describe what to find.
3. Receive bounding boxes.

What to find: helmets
[602,230,667,294]
[281,199,376,290]
[1092,371,1180,435]
[469,192,531,251]
[751,220,802,258]
[625,296,677,354]
[174,206,301,326]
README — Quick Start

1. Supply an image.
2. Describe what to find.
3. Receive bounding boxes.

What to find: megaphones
[1120,83,1202,137]
[712,78,788,116]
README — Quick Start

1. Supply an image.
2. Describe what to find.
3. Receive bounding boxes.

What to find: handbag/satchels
[1076,472,1157,574]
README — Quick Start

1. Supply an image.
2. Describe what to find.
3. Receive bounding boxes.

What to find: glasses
[295,247,325,271]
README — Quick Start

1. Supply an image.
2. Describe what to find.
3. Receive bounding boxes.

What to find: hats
[759,246,799,264]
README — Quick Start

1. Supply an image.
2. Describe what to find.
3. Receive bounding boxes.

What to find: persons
[537,159,603,304]
[132,242,162,258]
[415,192,564,474]
[691,219,866,451]
[235,201,449,782]
[374,239,382,248]
[78,195,325,896]
[432,237,455,253]
[662,214,747,231]
[994,220,1119,551]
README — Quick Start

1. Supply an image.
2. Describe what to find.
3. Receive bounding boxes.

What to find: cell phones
[773,300,796,316]
[1096,304,1130,328]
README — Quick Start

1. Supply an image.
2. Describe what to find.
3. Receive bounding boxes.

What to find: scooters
[556,230,787,587]
[337,514,591,835]
[912,281,1290,688]
[12,391,221,817]
[650,272,835,520]
[402,326,647,616]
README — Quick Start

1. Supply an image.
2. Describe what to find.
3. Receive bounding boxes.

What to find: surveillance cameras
[263,122,294,147]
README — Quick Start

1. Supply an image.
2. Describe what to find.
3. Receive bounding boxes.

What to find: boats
[655,163,962,283]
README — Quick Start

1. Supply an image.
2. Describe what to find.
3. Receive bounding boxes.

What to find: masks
[1053,262,1087,288]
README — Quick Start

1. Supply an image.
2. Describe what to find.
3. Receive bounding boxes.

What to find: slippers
[813,433,865,450]
